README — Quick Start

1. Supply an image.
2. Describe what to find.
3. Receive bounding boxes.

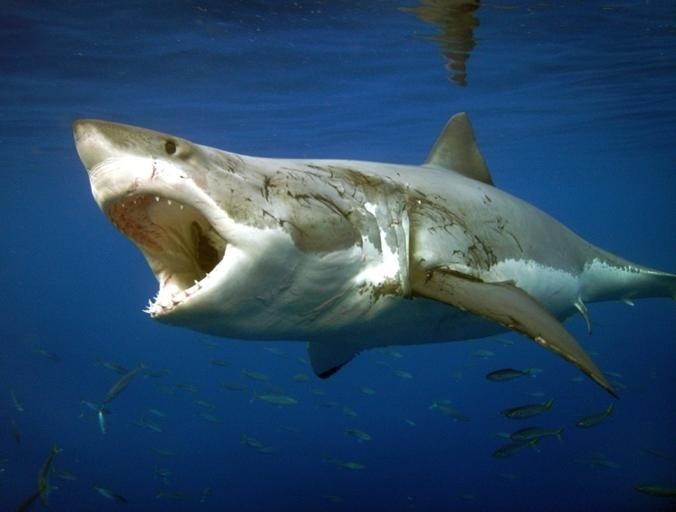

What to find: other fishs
[0,336,676,512]
